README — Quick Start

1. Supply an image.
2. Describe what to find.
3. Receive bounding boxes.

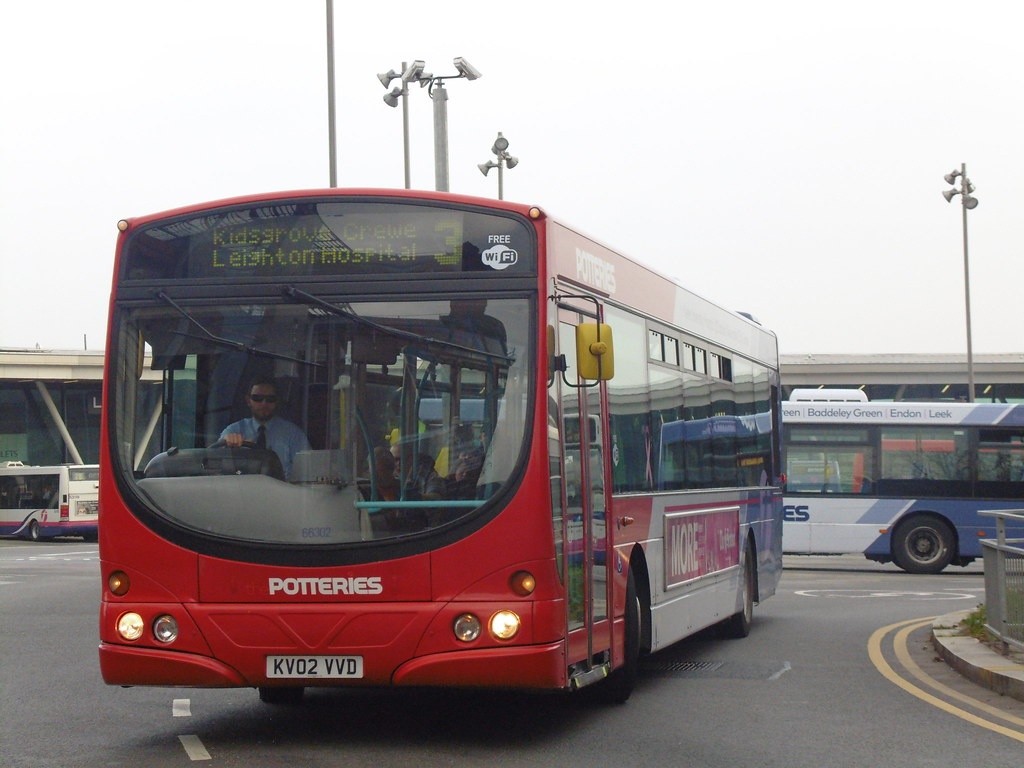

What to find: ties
[257,426,266,449]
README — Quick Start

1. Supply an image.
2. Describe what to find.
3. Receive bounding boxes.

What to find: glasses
[250,394,279,403]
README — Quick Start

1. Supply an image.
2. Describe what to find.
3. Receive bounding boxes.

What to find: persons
[214,375,313,484]
[370,422,495,529]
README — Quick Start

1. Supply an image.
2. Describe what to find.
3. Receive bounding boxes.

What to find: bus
[0,460,101,544]
[99,185,785,743]
[659,407,771,493]
[739,402,1024,572]
[552,411,619,561]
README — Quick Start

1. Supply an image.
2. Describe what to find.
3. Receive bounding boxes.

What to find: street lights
[476,129,519,196]
[943,163,978,398]
[373,57,482,197]
